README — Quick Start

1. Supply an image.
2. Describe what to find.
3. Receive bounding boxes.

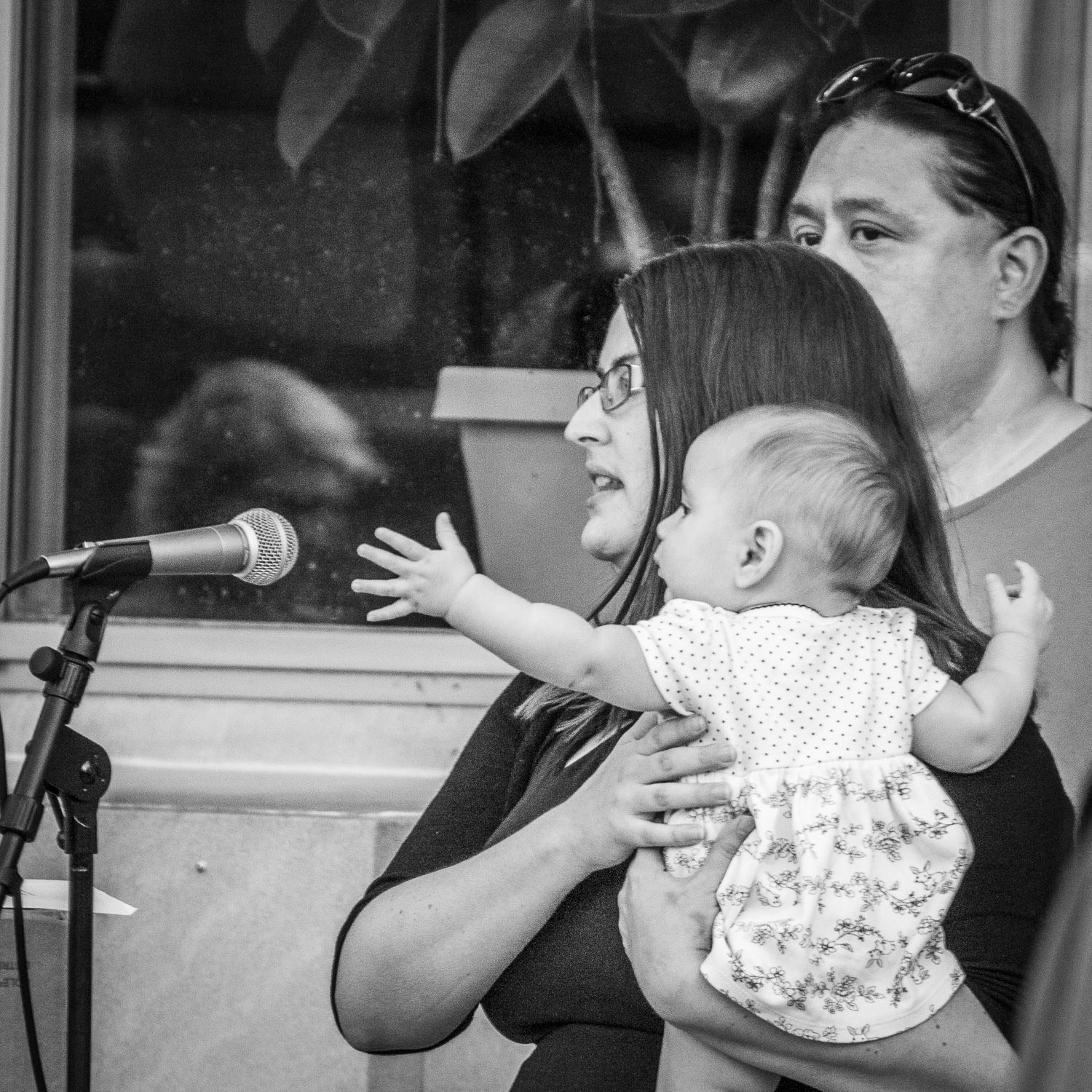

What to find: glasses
[817,52,1040,226]
[577,364,643,411]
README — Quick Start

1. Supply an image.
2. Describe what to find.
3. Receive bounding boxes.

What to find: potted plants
[248,2,886,611]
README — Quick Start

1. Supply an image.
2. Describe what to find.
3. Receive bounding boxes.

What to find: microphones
[3,508,300,594]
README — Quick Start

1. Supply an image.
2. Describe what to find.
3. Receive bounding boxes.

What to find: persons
[127,360,389,620]
[330,242,1079,1091]
[786,54,1092,815]
